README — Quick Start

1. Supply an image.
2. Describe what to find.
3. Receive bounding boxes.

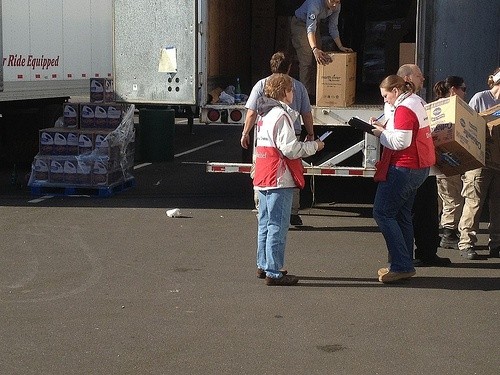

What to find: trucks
[2,1,500,200]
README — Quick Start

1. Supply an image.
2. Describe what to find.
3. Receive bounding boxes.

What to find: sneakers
[290,214,303,226]
[256,268,288,279]
[460,247,478,260]
[264,274,298,286]
[378,266,416,283]
[488,247,500,258]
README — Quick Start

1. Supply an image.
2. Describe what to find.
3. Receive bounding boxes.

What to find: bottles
[234,77,241,104]
[34,80,135,184]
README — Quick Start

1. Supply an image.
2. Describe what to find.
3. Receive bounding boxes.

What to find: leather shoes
[413,254,451,267]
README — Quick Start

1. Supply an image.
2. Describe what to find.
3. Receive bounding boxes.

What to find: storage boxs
[400,43,416,66]
[316,52,355,106]
[425,95,486,177]
[32,77,132,183]
[479,103,500,171]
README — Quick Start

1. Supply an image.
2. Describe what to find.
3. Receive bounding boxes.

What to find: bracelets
[312,47,318,52]
[242,131,248,137]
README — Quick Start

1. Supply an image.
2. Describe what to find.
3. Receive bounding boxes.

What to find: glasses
[458,86,466,92]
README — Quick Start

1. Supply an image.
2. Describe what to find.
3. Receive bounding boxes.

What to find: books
[348,116,383,138]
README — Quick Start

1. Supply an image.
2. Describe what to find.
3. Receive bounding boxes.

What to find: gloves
[312,47,333,66]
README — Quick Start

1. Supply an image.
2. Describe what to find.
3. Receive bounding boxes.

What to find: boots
[439,228,460,249]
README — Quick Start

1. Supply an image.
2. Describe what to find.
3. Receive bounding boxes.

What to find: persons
[457,67,500,259]
[367,63,451,282]
[240,52,315,226]
[434,76,465,249]
[289,0,352,104]
[254,73,324,286]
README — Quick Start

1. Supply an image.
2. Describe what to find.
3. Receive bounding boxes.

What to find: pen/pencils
[374,113,384,121]
[316,134,321,141]
